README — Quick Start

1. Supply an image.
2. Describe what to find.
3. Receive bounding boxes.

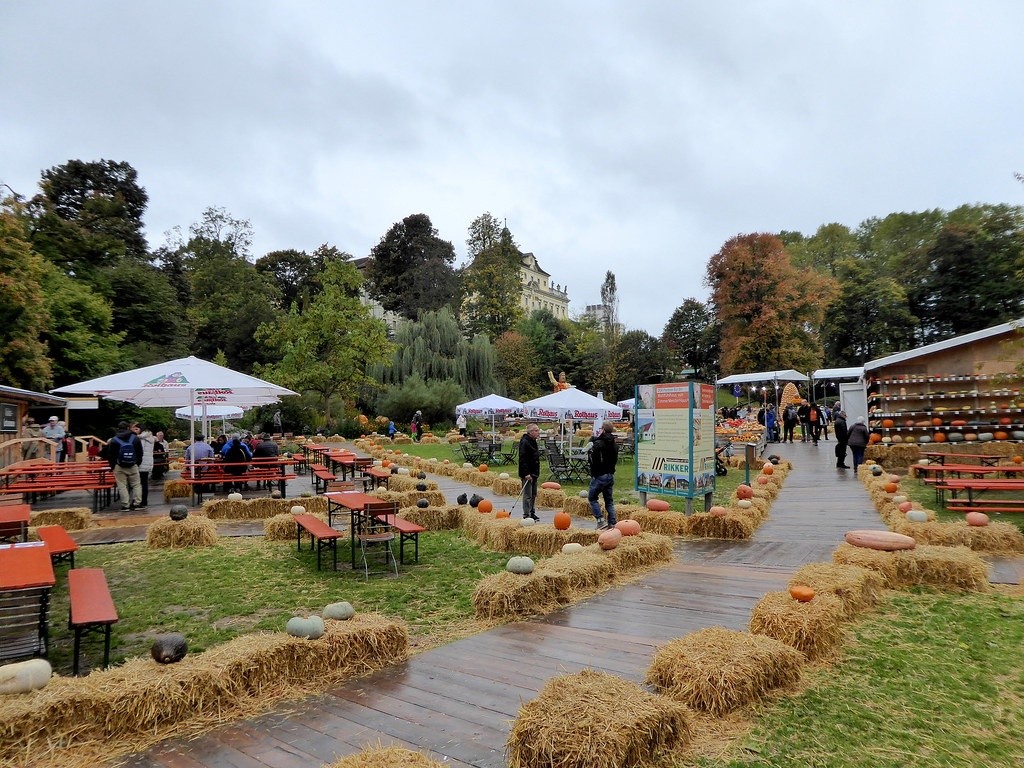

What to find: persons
[21,416,278,511]
[389,417,398,439]
[848,416,869,473]
[411,420,424,442]
[834,411,850,468]
[274,409,283,435]
[588,421,619,530]
[573,419,581,433]
[546,366,571,393]
[518,423,540,520]
[459,414,467,437]
[723,399,841,446]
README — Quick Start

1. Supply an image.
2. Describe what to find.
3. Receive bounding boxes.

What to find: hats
[49,416,58,421]
[261,433,272,440]
[820,406,825,411]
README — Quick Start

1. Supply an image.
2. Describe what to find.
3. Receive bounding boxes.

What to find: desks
[911,452,1024,506]
[472,443,489,463]
[566,455,586,480]
[0,445,392,664]
[561,447,584,454]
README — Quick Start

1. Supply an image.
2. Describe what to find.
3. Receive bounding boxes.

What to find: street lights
[774,386,780,423]
[747,387,757,407]
[823,380,836,407]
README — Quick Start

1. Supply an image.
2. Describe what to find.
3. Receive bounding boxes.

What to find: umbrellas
[617,397,635,411]
[455,393,524,459]
[523,387,623,476]
[48,356,301,477]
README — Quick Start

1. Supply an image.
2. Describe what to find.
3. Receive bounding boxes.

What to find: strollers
[717,443,732,474]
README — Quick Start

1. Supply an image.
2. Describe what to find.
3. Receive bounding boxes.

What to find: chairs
[351,458,374,493]
[449,419,637,485]
[361,501,399,580]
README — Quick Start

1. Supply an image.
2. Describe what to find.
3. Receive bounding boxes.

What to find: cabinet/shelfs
[867,374,1024,446]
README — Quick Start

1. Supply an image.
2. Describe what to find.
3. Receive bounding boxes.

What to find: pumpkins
[715,418,796,441]
[376,482,510,520]
[965,512,988,526]
[618,498,630,504]
[757,455,780,484]
[506,509,640,574]
[0,658,52,695]
[1013,455,1022,464]
[479,463,488,472]
[428,433,433,437]
[709,504,726,516]
[736,480,753,508]
[579,489,588,498]
[284,598,354,639]
[463,462,473,468]
[178,456,184,463]
[170,489,312,520]
[646,499,670,511]
[287,452,292,458]
[361,434,409,458]
[151,633,188,664]
[868,372,1024,444]
[541,482,561,489]
[866,459,927,522]
[789,585,816,603]
[382,456,449,479]
[499,472,509,480]
[844,530,916,551]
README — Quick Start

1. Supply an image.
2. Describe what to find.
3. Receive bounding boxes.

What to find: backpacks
[113,433,138,468]
[787,408,797,419]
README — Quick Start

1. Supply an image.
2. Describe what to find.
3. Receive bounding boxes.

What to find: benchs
[0,455,424,677]
[924,463,1024,513]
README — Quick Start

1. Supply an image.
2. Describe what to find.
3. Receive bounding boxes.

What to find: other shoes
[594,521,607,530]
[120,507,131,512]
[837,463,850,468]
[134,506,145,510]
[530,514,540,521]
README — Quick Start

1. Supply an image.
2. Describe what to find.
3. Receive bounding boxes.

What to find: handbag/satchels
[835,443,839,457]
